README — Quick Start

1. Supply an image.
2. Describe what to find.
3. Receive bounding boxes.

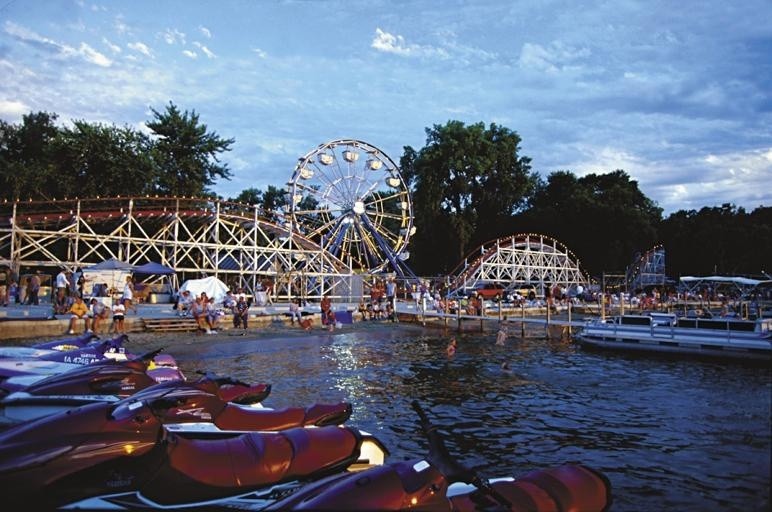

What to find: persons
[290,296,336,332]
[351,277,480,322]
[506,284,611,314]
[497,315,507,344]
[0,268,272,338]
[632,284,737,316]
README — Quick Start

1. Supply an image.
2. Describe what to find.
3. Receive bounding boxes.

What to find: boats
[576,308,772,358]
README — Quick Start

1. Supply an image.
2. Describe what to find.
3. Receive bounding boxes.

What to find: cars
[383,271,670,307]
